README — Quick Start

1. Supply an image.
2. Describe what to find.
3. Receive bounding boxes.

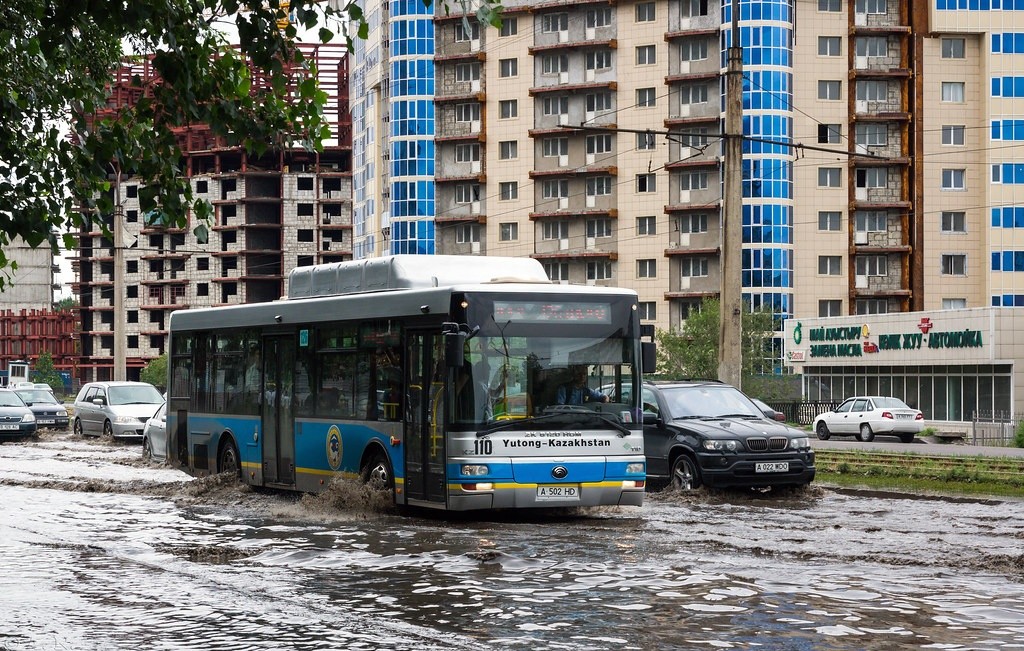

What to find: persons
[558,364,609,405]
[258,383,299,416]
[431,357,509,420]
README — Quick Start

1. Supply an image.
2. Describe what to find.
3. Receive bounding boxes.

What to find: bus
[167,254,656,518]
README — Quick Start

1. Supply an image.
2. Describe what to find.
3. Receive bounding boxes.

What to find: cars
[143,402,167,463]
[0,388,37,442]
[14,391,69,430]
[750,398,786,424]
[16,382,53,393]
[813,396,924,443]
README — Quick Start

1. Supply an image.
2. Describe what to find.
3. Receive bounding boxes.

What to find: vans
[73,381,166,442]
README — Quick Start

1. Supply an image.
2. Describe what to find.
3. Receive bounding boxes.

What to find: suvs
[595,378,816,498]
